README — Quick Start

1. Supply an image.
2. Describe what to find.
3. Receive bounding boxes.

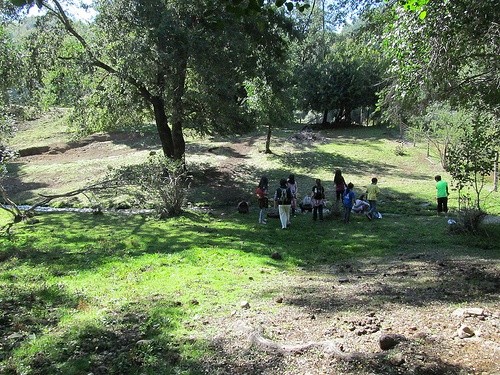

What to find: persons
[367,177,381,221]
[353,193,371,214]
[286,175,298,217]
[435,175,450,218]
[274,178,293,229]
[299,179,325,221]
[256,177,269,225]
[342,182,355,224]
[333,169,347,205]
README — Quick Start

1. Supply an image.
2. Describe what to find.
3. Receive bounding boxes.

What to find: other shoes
[366,212,372,219]
[262,218,267,225]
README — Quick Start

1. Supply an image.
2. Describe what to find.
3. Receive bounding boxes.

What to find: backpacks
[279,187,289,202]
